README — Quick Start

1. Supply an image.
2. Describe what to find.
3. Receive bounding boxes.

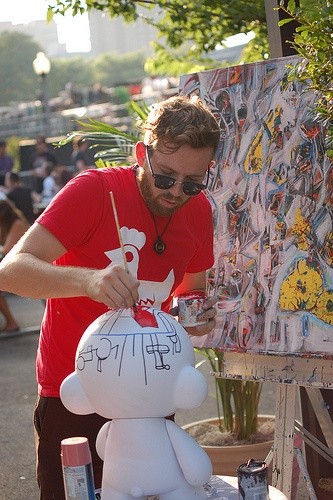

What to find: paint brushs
[107,188,141,317]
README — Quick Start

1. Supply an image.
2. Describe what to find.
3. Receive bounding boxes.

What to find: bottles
[60,437,98,500]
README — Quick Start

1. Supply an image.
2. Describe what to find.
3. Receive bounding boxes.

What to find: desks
[89,475,286,499]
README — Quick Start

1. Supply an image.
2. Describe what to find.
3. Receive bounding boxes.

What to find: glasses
[146,146,210,196]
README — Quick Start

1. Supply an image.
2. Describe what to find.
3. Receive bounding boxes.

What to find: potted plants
[181,346,275,479]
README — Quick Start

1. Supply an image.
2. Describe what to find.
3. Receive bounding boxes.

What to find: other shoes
[0,324,20,332]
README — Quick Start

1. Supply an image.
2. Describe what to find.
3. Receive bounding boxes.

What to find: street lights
[30,51,57,139]
